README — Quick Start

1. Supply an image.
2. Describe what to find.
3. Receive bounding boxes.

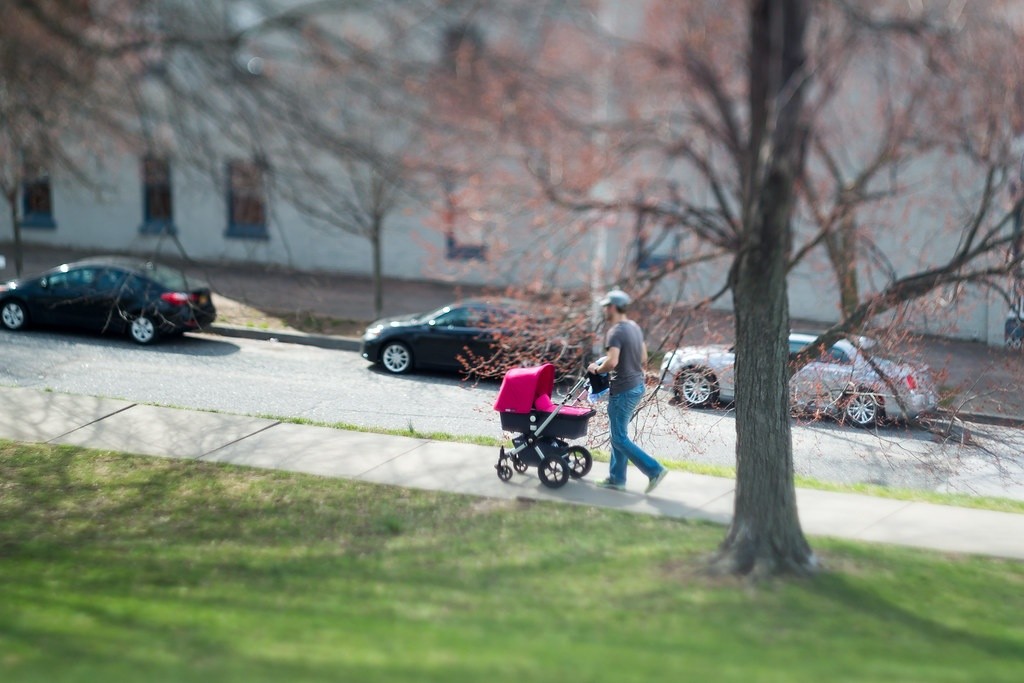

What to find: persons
[588,290,668,496]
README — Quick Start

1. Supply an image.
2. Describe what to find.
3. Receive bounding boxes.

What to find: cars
[361,295,594,387]
[658,327,938,430]
[0,255,217,346]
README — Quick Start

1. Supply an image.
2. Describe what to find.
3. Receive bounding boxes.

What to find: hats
[599,290,632,308]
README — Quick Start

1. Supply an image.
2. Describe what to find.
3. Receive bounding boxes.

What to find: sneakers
[595,478,625,490]
[645,467,669,494]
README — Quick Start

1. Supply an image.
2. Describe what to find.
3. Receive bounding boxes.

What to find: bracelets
[595,366,599,374]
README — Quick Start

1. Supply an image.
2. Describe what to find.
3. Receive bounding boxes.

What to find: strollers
[491,357,611,488]
